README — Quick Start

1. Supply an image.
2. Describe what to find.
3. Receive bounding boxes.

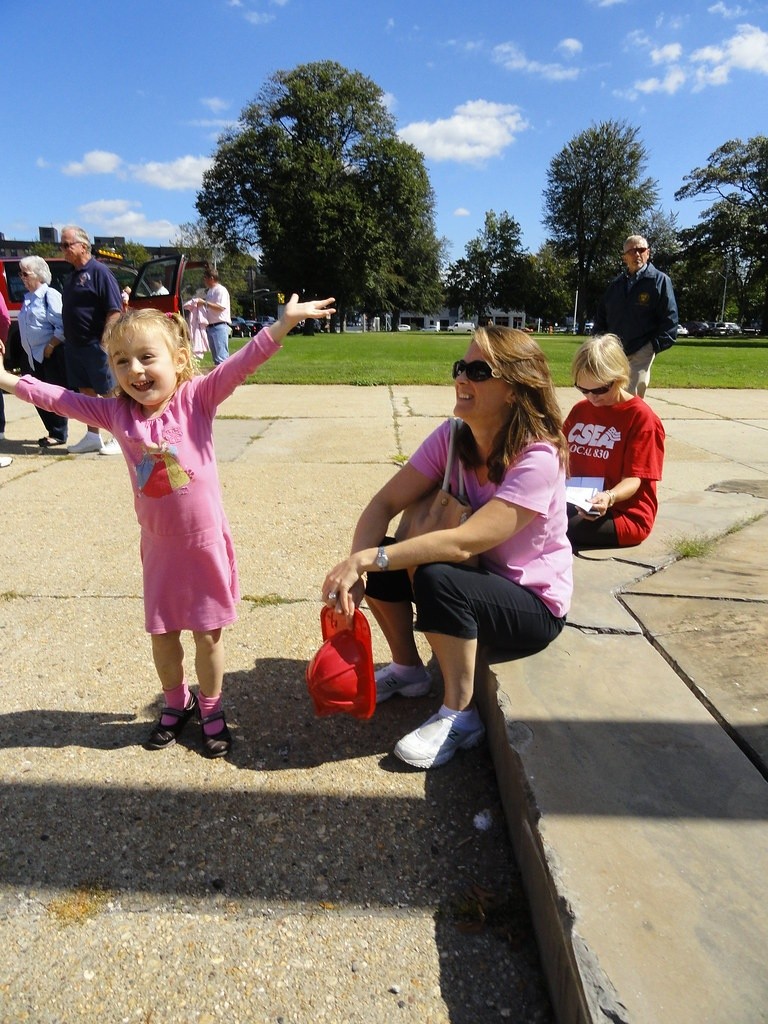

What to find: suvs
[0,255,189,374]
[447,322,475,333]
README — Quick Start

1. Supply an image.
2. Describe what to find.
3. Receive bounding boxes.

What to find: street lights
[707,271,727,323]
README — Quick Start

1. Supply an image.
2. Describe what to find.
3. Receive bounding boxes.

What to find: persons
[148,278,170,296]
[603,235,678,399]
[322,324,574,771]
[16,256,68,447]
[0,295,336,757]
[195,269,231,367]
[556,334,664,554]
[58,226,122,455]
[0,291,11,440]
[121,285,132,305]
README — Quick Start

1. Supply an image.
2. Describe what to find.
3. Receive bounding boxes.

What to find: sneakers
[394,706,484,769]
[67,433,105,453]
[374,665,434,705]
[99,437,123,454]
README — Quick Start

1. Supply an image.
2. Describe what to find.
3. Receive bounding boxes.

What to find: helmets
[305,604,376,719]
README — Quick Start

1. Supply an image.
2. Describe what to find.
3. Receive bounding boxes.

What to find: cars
[398,324,411,332]
[245,315,276,336]
[420,325,439,332]
[553,322,595,333]
[229,317,252,339]
[675,318,760,338]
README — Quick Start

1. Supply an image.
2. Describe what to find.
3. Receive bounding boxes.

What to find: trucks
[276,303,306,333]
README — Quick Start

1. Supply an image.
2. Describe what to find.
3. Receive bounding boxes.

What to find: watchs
[374,546,389,571]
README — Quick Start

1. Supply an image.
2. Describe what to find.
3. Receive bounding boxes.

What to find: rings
[329,593,336,599]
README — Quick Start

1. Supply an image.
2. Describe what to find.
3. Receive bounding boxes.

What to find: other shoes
[0,433,5,440]
[37,435,66,446]
[196,706,232,757]
[0,456,13,468]
[148,689,197,749]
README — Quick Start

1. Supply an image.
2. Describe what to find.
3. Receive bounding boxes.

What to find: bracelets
[49,344,54,349]
[606,490,615,508]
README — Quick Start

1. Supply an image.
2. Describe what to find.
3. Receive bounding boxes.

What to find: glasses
[452,358,510,382]
[624,247,648,255]
[60,242,83,250]
[574,379,615,394]
[19,271,34,278]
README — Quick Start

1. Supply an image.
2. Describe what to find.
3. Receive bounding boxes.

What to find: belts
[208,322,226,327]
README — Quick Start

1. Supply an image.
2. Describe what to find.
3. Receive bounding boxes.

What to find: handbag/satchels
[394,416,477,602]
